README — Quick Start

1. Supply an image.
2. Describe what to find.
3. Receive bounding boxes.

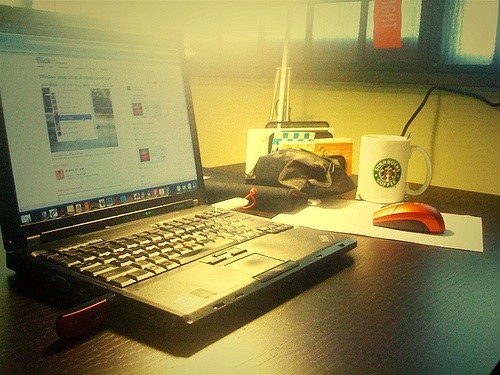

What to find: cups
[355,135,432,204]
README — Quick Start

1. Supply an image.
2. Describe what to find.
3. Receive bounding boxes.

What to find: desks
[0,163,499,374]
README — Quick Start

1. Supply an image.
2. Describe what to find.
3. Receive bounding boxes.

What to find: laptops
[1,5,358,335]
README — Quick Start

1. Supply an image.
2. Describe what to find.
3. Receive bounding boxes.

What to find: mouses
[372,199,447,236]
